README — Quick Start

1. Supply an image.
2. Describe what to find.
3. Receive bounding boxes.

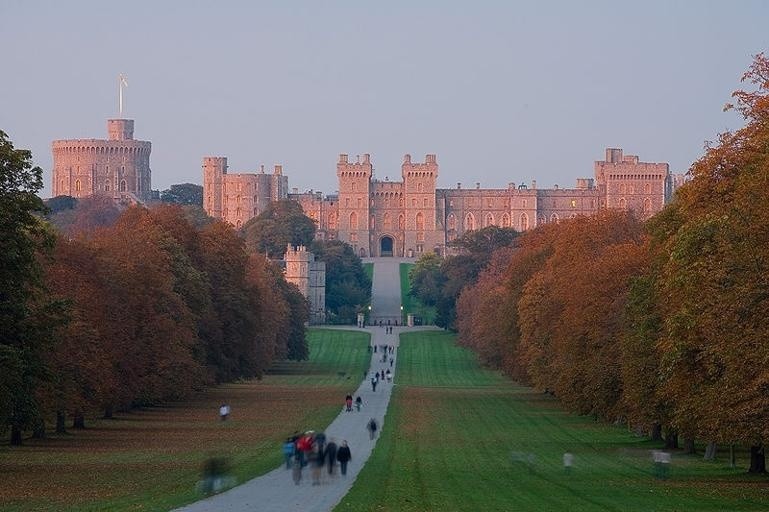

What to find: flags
[120,73,128,88]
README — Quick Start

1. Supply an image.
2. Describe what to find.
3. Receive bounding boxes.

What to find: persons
[367,417,378,439]
[346,343,395,411]
[219,403,230,419]
[282,429,350,486]
